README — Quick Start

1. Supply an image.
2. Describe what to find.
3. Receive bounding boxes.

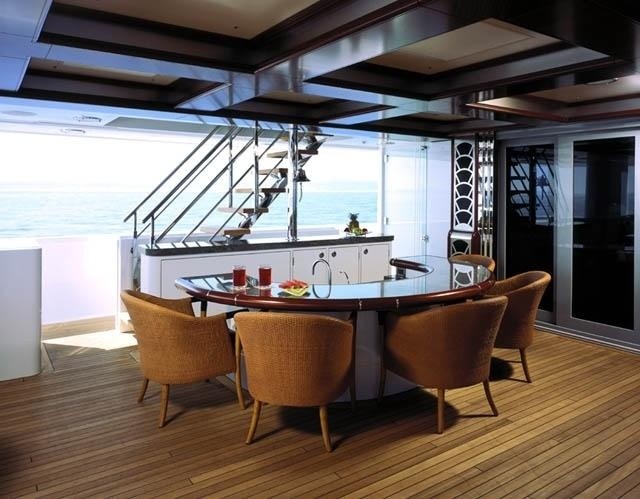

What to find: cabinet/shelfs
[360,243,391,284]
[291,245,360,286]
[139,253,290,329]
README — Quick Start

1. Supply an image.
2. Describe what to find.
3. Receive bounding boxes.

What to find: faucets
[311,258,332,285]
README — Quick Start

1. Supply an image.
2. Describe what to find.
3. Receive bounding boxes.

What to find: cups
[232,265,247,289]
[257,263,272,290]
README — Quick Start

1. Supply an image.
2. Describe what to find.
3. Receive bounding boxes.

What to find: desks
[175,255,496,404]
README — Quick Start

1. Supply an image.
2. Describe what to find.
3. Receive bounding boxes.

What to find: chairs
[482,270,552,383]
[120,289,249,428]
[378,295,509,434]
[449,254,496,272]
[233,311,357,453]
